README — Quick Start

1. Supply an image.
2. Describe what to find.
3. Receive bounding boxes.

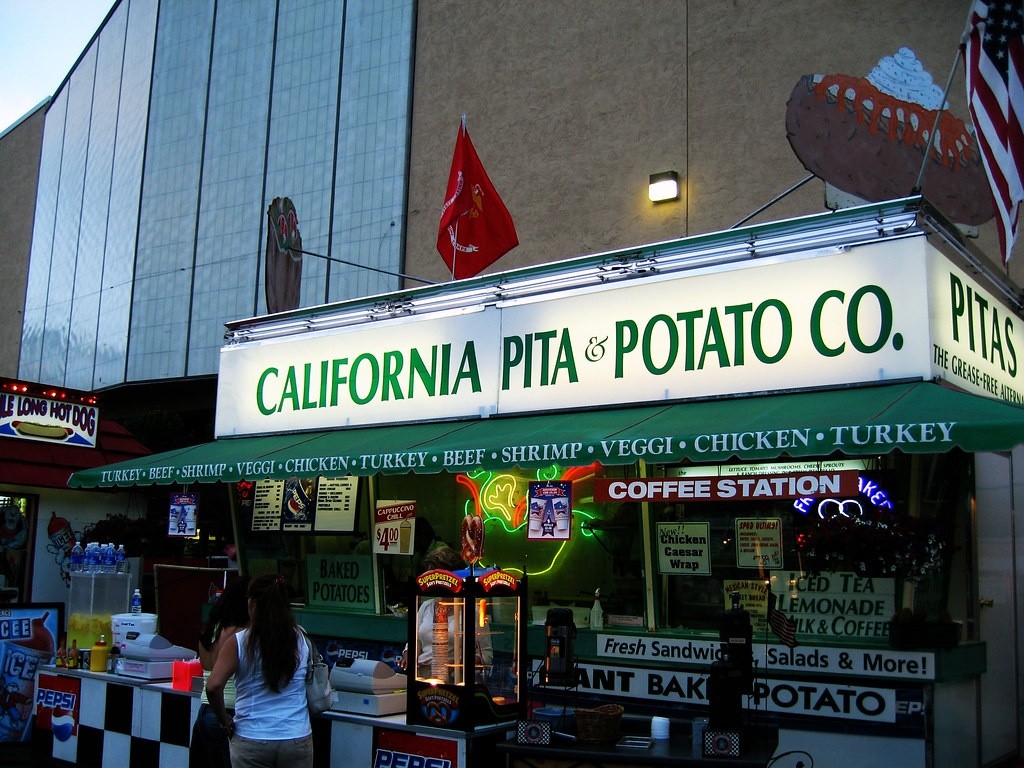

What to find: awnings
[66,381,1024,489]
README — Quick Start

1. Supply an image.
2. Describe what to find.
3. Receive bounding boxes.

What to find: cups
[400,521,411,553]
[431,601,449,682]
[283,478,314,520]
[78,649,91,669]
[0,640,55,742]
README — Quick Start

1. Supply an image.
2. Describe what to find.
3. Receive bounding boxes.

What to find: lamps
[648,171,681,205]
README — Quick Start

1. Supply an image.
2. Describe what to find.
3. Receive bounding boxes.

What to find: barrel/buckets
[110,613,157,646]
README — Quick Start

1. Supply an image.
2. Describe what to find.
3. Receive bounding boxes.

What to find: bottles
[56,638,67,667]
[68,640,80,669]
[70,542,127,575]
[545,607,575,685]
[718,592,753,657]
[131,589,142,616]
[107,647,121,673]
[90,642,108,671]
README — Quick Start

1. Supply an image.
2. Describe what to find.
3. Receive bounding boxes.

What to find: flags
[959,0,1024,265]
[437,117,519,279]
[769,608,799,647]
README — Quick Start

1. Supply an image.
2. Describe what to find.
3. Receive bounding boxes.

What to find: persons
[412,517,468,576]
[189,576,250,768]
[206,575,313,768]
[397,549,492,681]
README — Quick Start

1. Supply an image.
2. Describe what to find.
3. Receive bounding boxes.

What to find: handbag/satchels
[296,624,334,714]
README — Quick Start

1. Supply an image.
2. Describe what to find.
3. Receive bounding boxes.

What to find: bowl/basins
[532,707,575,729]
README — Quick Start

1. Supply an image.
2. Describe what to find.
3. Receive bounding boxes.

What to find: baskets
[574,704,624,745]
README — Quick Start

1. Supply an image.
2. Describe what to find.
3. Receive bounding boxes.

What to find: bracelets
[220,714,232,729]
